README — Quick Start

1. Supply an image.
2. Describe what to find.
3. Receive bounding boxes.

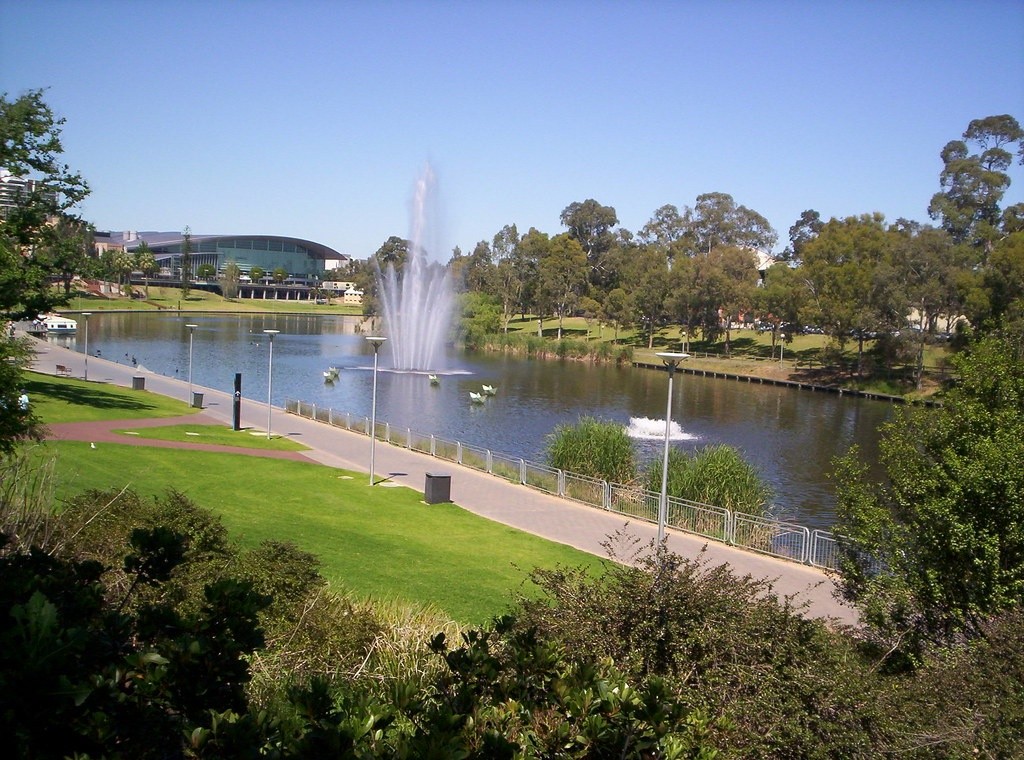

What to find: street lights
[654,352,691,561]
[81,312,92,381]
[263,329,280,440]
[186,324,197,409]
[365,336,387,486]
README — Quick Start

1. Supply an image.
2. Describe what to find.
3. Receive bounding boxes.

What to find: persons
[18,389,29,423]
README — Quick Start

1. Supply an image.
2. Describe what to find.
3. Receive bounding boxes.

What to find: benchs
[56,364,72,378]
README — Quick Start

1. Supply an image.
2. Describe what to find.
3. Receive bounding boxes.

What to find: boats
[18,313,78,334]
[323,366,340,382]
[482,384,498,396]
[427,373,441,384]
[469,391,488,404]
